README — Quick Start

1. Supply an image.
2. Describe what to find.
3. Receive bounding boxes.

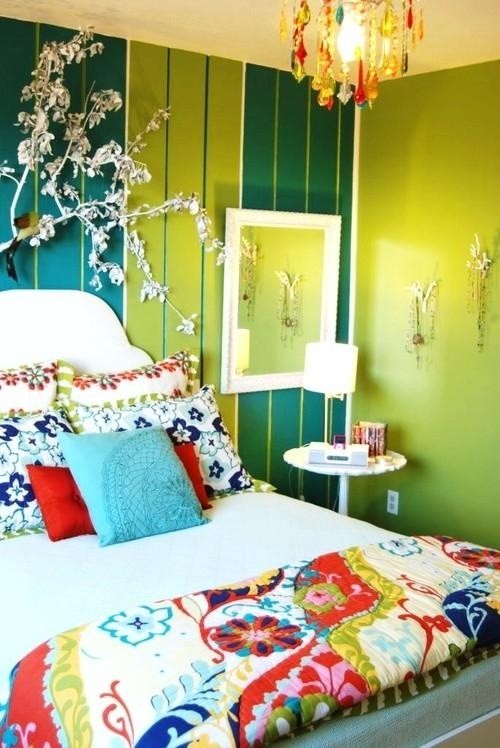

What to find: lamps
[237,319,254,376]
[301,339,358,466]
[271,0,424,109]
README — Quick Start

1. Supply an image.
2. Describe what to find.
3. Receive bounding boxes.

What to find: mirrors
[216,204,344,399]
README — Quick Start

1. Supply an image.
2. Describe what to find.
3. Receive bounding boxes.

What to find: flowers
[1,19,239,339]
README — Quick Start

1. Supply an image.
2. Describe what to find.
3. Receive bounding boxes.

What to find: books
[351,418,389,459]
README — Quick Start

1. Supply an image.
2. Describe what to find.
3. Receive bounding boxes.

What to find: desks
[282,446,409,520]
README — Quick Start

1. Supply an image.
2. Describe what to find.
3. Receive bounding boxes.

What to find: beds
[0,286,500,747]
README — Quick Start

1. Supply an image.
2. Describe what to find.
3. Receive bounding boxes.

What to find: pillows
[59,347,195,412]
[25,442,214,543]
[0,353,71,409]
[55,381,275,505]
[56,426,214,547]
[0,403,78,545]
